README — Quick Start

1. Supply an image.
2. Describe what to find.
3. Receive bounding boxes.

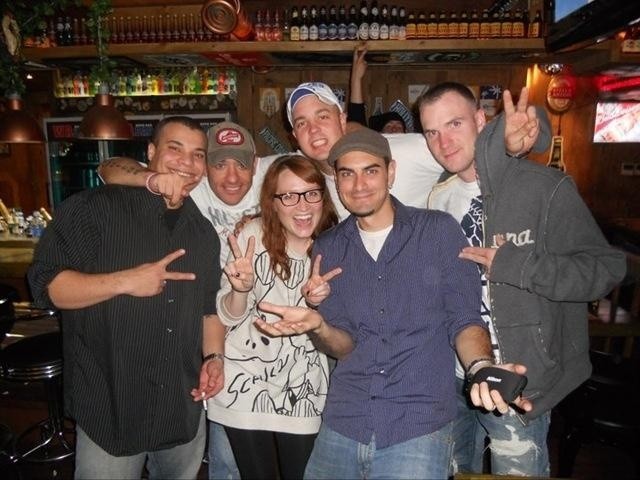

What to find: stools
[0,330,78,467]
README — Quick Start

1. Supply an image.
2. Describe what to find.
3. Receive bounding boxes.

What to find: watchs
[202,351,222,362]
[466,359,491,375]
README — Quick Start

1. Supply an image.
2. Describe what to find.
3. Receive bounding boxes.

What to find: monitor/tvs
[543,0,640,54]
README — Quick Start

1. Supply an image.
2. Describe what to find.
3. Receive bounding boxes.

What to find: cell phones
[470,366,529,403]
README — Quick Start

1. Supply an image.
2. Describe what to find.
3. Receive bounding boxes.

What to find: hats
[285,81,344,128]
[327,128,392,167]
[205,121,257,169]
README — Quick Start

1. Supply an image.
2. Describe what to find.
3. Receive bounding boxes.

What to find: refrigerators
[41,111,230,214]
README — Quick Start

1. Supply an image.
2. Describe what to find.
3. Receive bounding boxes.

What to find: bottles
[531,10,542,38]
[428,14,438,39]
[111,15,118,44]
[290,6,298,41]
[274,6,282,42]
[512,12,524,38]
[181,13,188,40]
[126,15,132,43]
[182,69,236,94]
[196,12,203,42]
[55,12,64,45]
[82,18,90,44]
[468,13,479,38]
[256,7,265,40]
[373,97,384,114]
[135,15,140,42]
[405,13,416,41]
[360,0,369,40]
[346,5,357,41]
[71,17,81,45]
[205,29,230,42]
[110,72,143,94]
[300,8,308,41]
[157,75,165,91]
[173,12,179,42]
[147,75,153,93]
[141,15,149,42]
[490,13,501,38]
[458,13,468,38]
[338,4,345,41]
[265,8,273,41]
[479,12,490,37]
[448,12,458,38]
[501,13,513,38]
[380,4,388,40]
[438,13,448,39]
[546,137,566,173]
[150,15,158,41]
[55,70,99,96]
[417,14,427,39]
[66,17,74,44]
[101,16,110,43]
[156,14,165,42]
[188,12,195,42]
[522,10,530,38]
[369,0,379,38]
[318,6,327,41]
[399,7,406,41]
[329,7,338,40]
[388,5,398,39]
[309,8,318,40]
[165,13,172,41]
[119,15,126,45]
[153,74,158,91]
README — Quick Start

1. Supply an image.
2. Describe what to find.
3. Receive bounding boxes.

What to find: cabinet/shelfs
[17,1,547,67]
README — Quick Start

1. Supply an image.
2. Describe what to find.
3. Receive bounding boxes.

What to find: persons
[347,40,404,135]
[417,82,628,480]
[255,130,532,479]
[206,156,339,480]
[281,82,538,220]
[94,121,313,480]
[27,116,226,480]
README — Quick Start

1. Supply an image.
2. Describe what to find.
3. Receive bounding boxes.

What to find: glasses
[274,187,325,207]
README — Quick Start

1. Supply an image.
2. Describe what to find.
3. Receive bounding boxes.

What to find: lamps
[74,1,135,143]
[0,68,47,145]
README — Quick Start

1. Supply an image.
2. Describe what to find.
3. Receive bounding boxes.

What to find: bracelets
[144,172,165,197]
